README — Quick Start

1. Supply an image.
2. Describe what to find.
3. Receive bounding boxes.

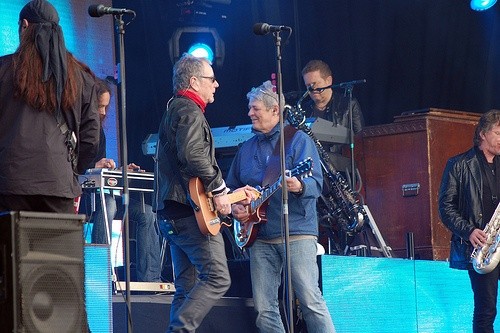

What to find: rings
[222,208,226,211]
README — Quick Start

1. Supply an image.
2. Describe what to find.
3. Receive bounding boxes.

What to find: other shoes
[166,320,188,333]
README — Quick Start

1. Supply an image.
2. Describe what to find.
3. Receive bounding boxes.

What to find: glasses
[195,75,215,82]
[252,87,279,104]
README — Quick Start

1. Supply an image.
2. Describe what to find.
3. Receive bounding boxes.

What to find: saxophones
[470,205,496,274]
[283,83,368,236]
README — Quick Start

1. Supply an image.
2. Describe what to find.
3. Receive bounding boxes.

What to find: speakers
[0,211,88,333]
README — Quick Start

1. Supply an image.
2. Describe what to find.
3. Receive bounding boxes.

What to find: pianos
[76,160,156,192]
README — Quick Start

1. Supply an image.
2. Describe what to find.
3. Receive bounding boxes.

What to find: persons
[293,59,366,202]
[154,55,232,333]
[79,78,170,295]
[225,80,338,333]
[438,109,500,333]
[0,0,100,333]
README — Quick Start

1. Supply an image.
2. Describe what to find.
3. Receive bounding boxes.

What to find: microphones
[88,4,134,17]
[253,23,290,35]
[298,84,315,104]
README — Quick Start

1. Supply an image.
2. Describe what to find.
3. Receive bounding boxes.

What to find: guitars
[232,158,313,248]
[189,174,260,236]
